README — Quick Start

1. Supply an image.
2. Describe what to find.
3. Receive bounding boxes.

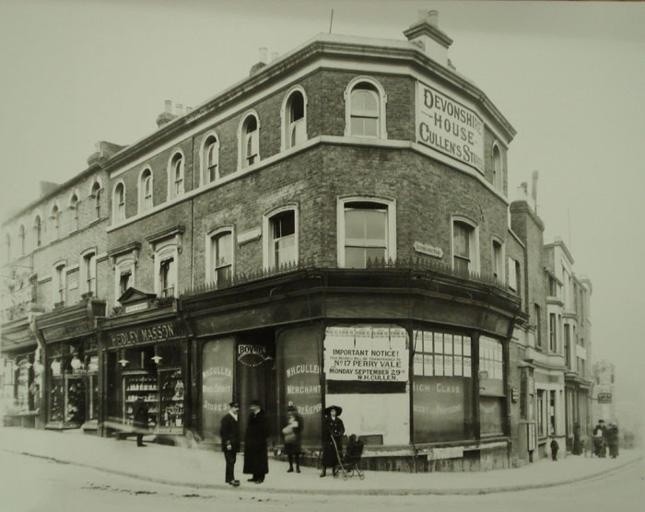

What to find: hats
[230,401,240,407]
[324,406,342,416]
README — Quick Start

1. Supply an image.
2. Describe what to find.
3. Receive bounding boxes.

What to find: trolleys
[328,433,365,482]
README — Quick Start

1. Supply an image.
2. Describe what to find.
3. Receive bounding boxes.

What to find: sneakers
[228,469,337,487]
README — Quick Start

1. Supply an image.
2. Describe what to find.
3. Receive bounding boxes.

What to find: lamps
[118,349,130,367]
[70,355,82,369]
[150,345,164,363]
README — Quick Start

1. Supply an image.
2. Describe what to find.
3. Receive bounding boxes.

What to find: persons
[131,393,150,446]
[571,420,636,459]
[550,437,559,461]
[319,405,345,476]
[219,402,241,486]
[243,401,268,483]
[281,406,304,473]
